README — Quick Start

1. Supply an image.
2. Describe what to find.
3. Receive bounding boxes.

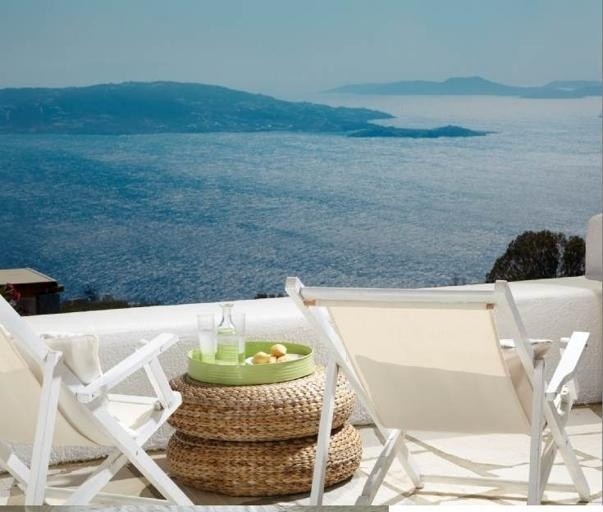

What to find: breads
[251,351,271,365]
[271,345,287,357]
[277,355,288,363]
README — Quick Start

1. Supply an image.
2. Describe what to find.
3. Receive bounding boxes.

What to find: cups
[232,311,246,366]
[196,312,217,363]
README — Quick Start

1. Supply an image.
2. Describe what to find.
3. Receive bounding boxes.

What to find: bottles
[216,302,239,365]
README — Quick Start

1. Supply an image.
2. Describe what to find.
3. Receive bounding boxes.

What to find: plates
[245,354,307,366]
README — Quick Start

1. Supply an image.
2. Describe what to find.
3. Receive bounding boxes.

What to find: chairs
[1,293,191,510]
[280,273,592,505]
